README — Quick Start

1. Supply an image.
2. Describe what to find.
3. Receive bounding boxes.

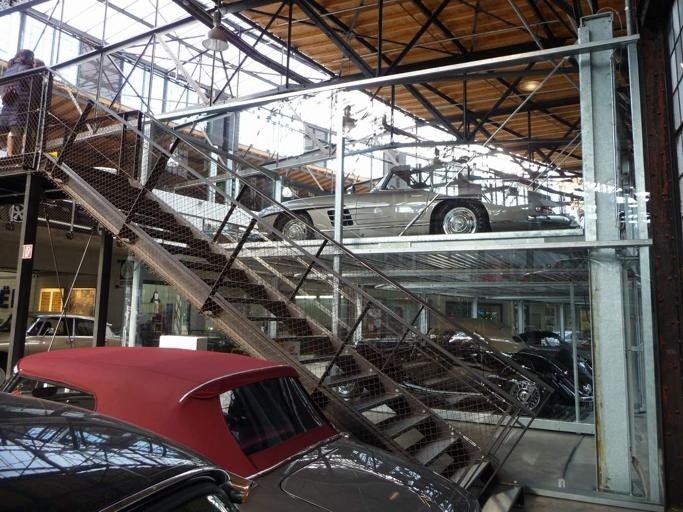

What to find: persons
[0,49,35,155]
[29,59,48,151]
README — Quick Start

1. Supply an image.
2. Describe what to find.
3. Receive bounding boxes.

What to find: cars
[516,254,641,282]
[1,390,235,512]
[1,311,122,371]
[16,346,480,512]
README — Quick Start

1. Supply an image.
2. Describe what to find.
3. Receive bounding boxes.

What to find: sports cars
[247,161,580,242]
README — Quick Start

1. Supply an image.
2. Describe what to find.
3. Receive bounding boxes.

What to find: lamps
[202,8,228,52]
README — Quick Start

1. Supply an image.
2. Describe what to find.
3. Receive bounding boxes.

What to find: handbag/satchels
[0,87,16,106]
